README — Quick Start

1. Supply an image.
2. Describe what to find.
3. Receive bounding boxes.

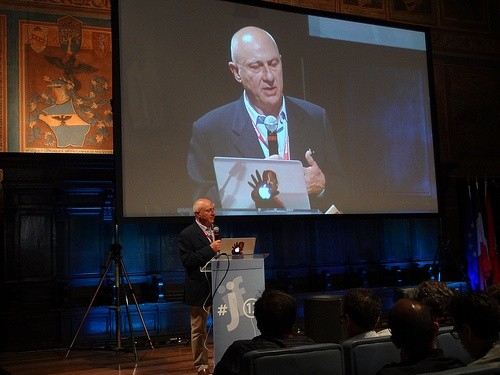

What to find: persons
[213,289,318,375]
[338,290,392,345]
[458,283,500,367]
[186,24,356,213]
[413,280,458,328]
[376,297,467,375]
[178,198,222,375]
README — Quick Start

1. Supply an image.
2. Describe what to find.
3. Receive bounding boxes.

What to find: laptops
[218,238,256,255]
[213,157,310,211]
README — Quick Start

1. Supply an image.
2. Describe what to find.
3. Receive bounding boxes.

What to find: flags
[466,179,500,294]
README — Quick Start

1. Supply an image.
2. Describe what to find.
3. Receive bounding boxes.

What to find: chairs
[239,324,500,374]
[266,264,438,295]
[65,280,213,349]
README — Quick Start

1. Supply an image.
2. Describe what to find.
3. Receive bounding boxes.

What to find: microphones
[214,227,220,258]
[264,115,278,156]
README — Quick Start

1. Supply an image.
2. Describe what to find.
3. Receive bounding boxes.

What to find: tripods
[64,224,154,363]
